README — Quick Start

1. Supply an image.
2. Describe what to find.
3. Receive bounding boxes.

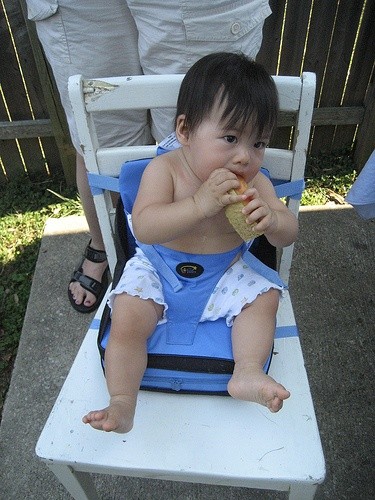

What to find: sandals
[67,238,109,313]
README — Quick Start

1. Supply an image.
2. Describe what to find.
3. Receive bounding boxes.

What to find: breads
[222,175,265,244]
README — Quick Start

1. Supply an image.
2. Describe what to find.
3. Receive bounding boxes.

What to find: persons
[26,0,272,314]
[82,53,300,434]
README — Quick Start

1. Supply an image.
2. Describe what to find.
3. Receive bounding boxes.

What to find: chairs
[35,72,326,500]
[85,130,304,396]
